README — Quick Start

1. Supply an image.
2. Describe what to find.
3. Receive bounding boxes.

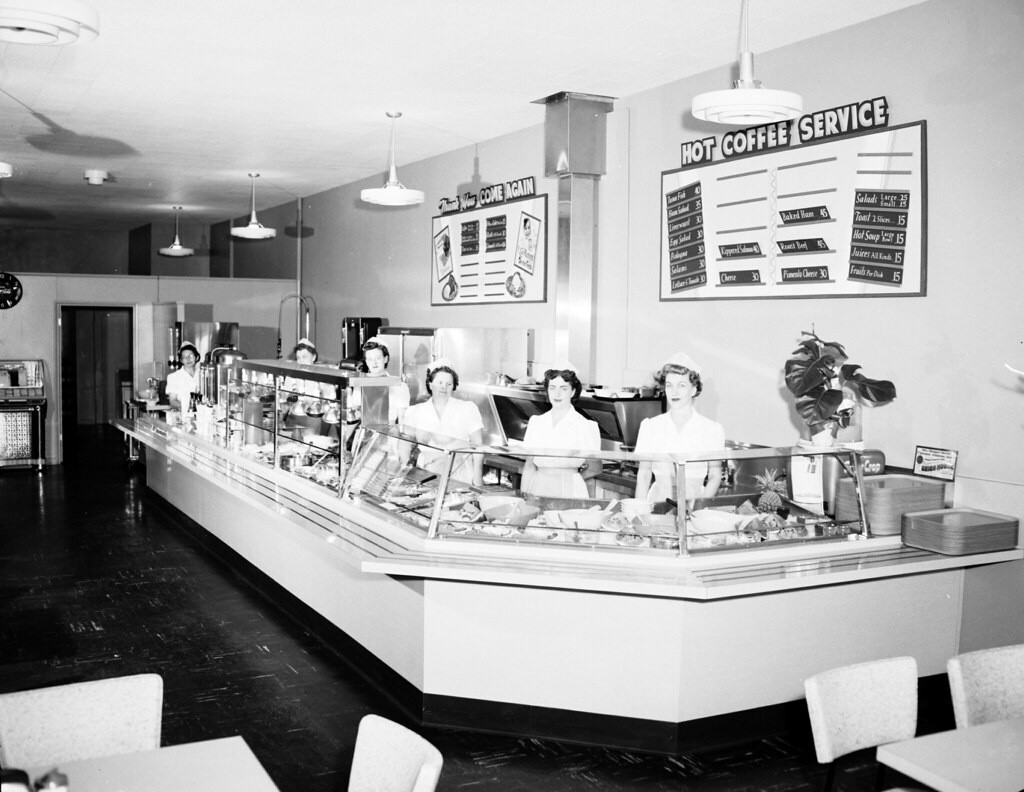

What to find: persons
[282,338,336,404]
[633,354,724,503]
[519,359,603,499]
[361,337,486,489]
[162,340,204,412]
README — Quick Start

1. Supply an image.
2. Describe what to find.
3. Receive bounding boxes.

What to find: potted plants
[784,323,896,444]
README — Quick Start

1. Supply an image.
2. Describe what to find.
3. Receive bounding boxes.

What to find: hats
[426,358,460,381]
[297,338,315,349]
[549,359,579,380]
[661,352,703,386]
[368,336,389,353]
[180,341,197,350]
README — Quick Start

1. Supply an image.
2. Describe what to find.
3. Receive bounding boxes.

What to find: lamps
[361,112,425,206]
[692,0,803,125]
[230,173,276,239]
[159,206,194,256]
[0,0,100,46]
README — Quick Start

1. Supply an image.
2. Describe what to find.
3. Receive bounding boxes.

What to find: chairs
[947,643,1024,729]
[803,656,919,792]
[0,673,164,770]
[348,713,444,792]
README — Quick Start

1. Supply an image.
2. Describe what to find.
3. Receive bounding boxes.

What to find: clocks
[0,272,23,309]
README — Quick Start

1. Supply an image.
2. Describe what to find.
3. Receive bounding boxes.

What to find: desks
[0,735,282,792]
[876,715,1024,792]
[0,398,48,477]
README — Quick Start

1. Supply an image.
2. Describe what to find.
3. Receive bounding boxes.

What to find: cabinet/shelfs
[225,359,401,500]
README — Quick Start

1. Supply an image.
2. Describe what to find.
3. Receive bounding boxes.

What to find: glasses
[181,353,195,360]
